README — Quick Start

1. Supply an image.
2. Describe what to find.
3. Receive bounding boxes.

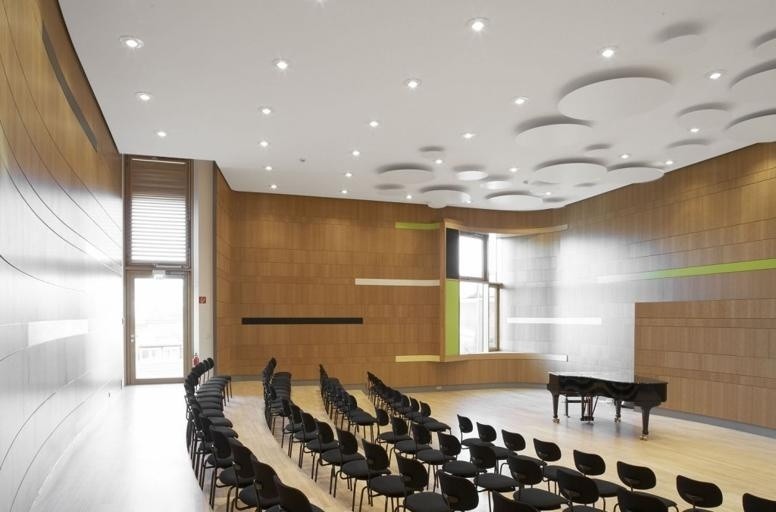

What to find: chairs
[401,393,410,415]
[613,461,678,512]
[231,443,280,512]
[556,469,608,512]
[366,371,401,421]
[617,486,667,512]
[333,431,391,512]
[362,441,415,512]
[409,397,438,423]
[500,430,550,492]
[436,432,492,512]
[573,449,627,510]
[439,471,479,512]
[195,418,244,489]
[470,422,517,473]
[289,414,338,480]
[457,415,479,449]
[317,363,356,438]
[190,408,238,472]
[411,423,457,490]
[742,493,776,512]
[375,410,413,453]
[676,475,723,512]
[533,438,573,496]
[287,405,317,457]
[314,422,364,497]
[187,408,232,460]
[506,454,569,512]
[394,452,452,512]
[389,416,432,468]
[492,492,539,512]
[253,479,283,512]
[260,357,293,434]
[275,482,325,512]
[202,431,255,508]
[179,356,234,415]
[349,395,376,441]
[469,443,516,512]
[420,402,452,435]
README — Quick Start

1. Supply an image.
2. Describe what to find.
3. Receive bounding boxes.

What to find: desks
[546,370,669,441]
